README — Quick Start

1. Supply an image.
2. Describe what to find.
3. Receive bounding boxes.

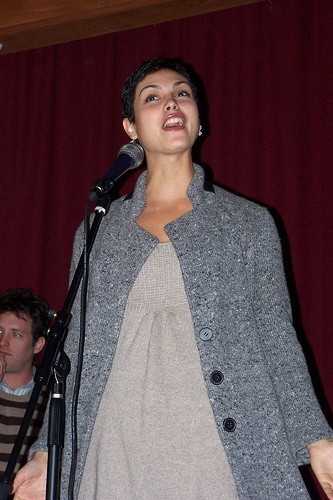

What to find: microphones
[88,142,144,201]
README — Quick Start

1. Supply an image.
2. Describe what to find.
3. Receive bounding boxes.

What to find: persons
[8,55,333,500]
[0,286,53,500]
